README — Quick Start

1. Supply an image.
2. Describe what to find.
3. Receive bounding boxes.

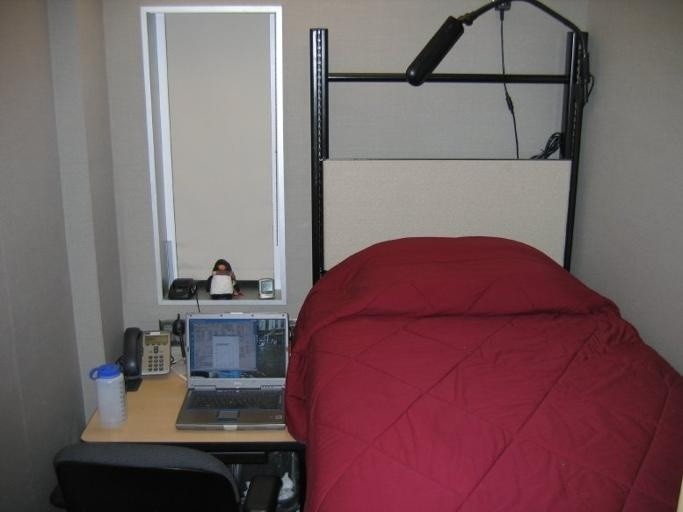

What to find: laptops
[176,312,289,433]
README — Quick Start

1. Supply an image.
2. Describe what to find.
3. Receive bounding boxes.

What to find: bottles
[90,364,127,427]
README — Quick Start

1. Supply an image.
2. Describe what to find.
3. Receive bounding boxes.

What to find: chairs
[48,441,282,512]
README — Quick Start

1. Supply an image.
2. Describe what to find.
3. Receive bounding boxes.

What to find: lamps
[404,0,595,160]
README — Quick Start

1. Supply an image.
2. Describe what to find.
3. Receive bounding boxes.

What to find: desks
[78,364,307,511]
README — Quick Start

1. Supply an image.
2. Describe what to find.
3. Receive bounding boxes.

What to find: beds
[281,27,681,512]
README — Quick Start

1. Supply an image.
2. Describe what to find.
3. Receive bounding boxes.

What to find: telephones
[123,327,171,376]
